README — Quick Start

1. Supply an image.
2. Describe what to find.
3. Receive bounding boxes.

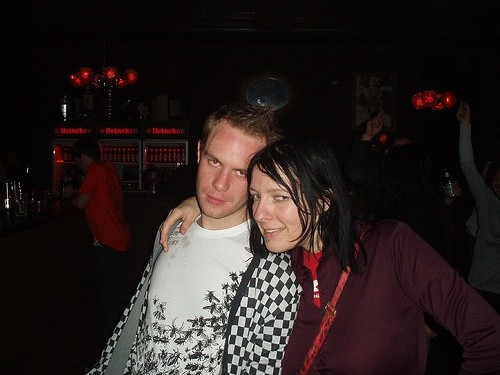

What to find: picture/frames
[352,70,397,132]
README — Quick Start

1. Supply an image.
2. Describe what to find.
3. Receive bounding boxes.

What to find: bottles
[444,168,457,198]
[102,143,186,162]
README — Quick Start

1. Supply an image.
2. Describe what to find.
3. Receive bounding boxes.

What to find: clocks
[238,70,297,116]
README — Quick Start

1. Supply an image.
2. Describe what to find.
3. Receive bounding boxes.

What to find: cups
[14,199,27,216]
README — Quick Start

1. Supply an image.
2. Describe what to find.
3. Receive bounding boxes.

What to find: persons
[456,100,500,316]
[326,66,390,169]
[246,134,500,375]
[67,133,137,363]
[335,108,459,246]
[88,100,307,375]
